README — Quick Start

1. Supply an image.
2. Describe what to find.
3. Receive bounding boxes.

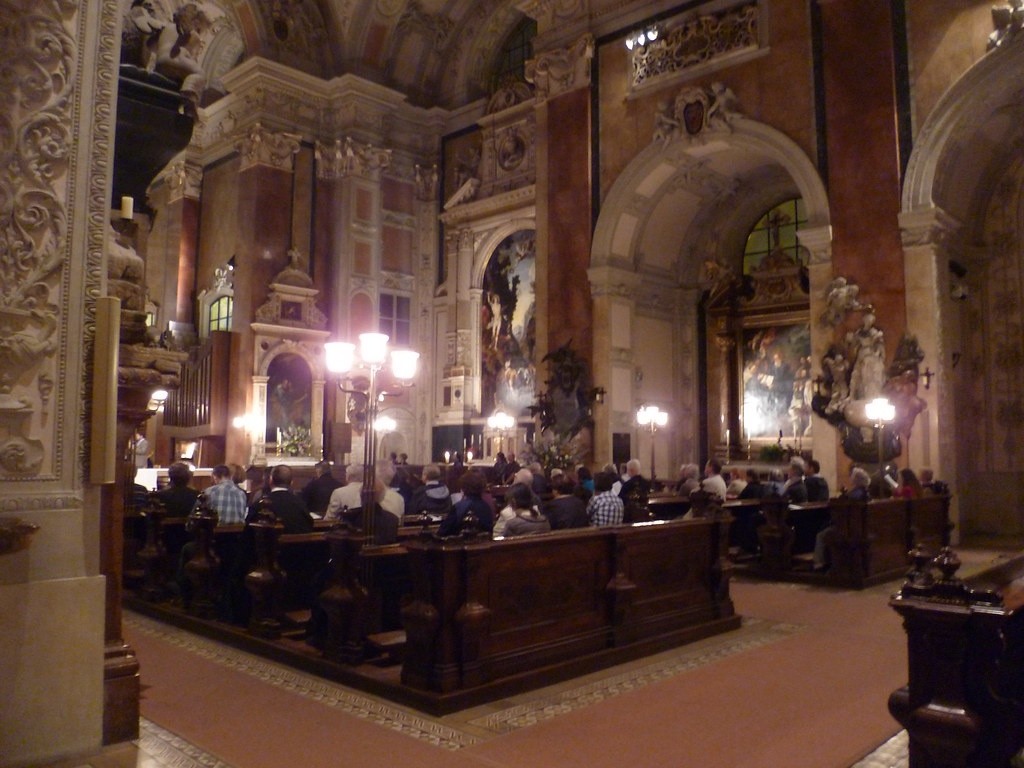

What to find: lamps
[488,412,514,452]
[637,406,668,490]
[325,332,420,665]
[146,390,169,414]
[864,398,895,497]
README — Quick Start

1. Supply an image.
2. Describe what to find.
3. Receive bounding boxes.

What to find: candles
[747,430,752,445]
[320,433,324,449]
[444,433,482,464]
[277,427,283,447]
[726,429,731,447]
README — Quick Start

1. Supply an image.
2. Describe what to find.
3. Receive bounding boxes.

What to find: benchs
[645,479,952,588]
[123,502,745,718]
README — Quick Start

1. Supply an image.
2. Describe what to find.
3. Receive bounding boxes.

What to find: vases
[290,443,300,456]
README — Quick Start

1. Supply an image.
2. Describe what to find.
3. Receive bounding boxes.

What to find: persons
[708,81,741,131]
[672,456,933,570]
[846,314,886,402]
[132,451,650,540]
[652,100,681,147]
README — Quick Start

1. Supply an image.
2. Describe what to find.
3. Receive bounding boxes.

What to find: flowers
[278,425,311,456]
[521,432,592,472]
